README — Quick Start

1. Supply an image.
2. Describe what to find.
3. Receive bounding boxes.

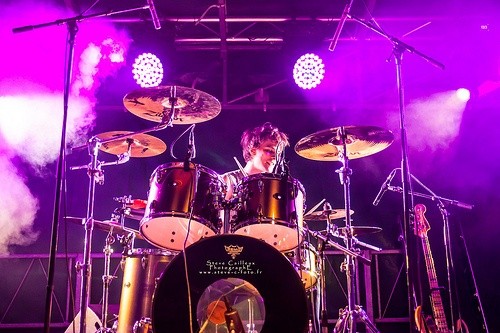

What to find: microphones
[328,0,353,52]
[275,151,283,174]
[373,169,396,206]
[147,0,161,30]
[188,131,196,158]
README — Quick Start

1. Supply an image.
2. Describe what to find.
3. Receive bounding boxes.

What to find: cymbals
[93,131,167,158]
[63,217,144,240]
[122,85,222,124]
[303,208,354,221]
[318,226,382,236]
[293,126,395,161]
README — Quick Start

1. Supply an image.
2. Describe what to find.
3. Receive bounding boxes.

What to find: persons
[220,121,291,235]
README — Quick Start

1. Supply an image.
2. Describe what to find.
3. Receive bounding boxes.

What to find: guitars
[413,204,470,333]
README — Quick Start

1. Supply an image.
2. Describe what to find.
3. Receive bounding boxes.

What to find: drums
[284,240,322,295]
[139,161,227,252]
[114,248,182,333]
[152,233,316,333]
[229,172,306,253]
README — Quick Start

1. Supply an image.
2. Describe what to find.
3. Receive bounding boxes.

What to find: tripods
[331,138,381,333]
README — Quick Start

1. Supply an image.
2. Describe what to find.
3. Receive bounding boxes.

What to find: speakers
[64,306,102,333]
